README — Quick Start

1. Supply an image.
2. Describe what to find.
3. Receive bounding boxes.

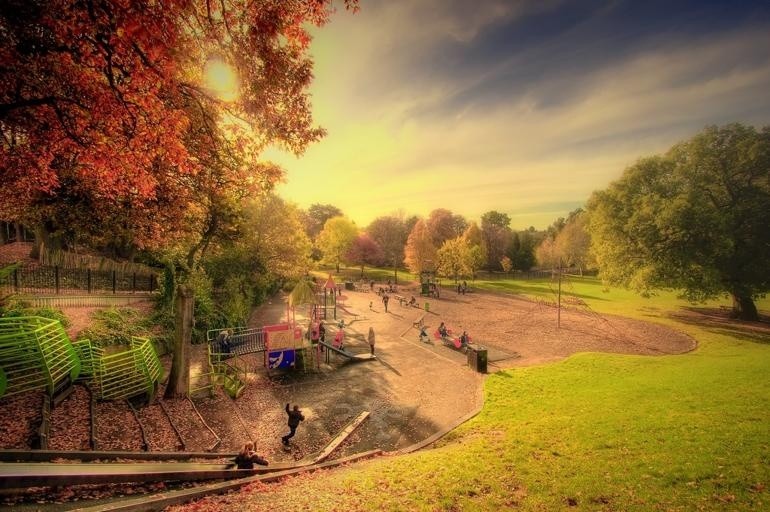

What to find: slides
[321,340,376,360]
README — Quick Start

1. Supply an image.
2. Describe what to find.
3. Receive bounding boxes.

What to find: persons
[381,291,389,313]
[458,280,467,294]
[406,296,416,306]
[217,328,233,358]
[320,320,325,352]
[420,322,468,347]
[282,403,305,445]
[235,440,269,475]
[368,326,375,355]
